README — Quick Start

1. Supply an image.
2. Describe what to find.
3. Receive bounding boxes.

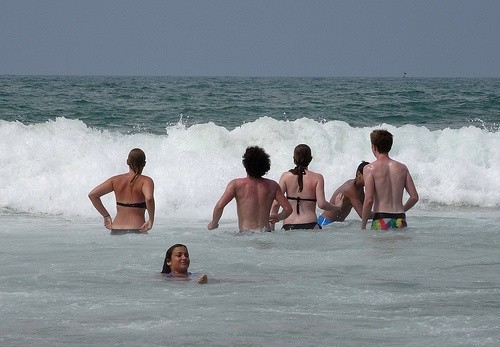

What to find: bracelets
[104,214,109,218]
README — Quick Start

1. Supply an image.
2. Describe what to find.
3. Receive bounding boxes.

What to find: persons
[161,244,207,284]
[318,160,374,226]
[207,145,293,232]
[88,148,154,233]
[270,144,344,231]
[362,129,418,230]
